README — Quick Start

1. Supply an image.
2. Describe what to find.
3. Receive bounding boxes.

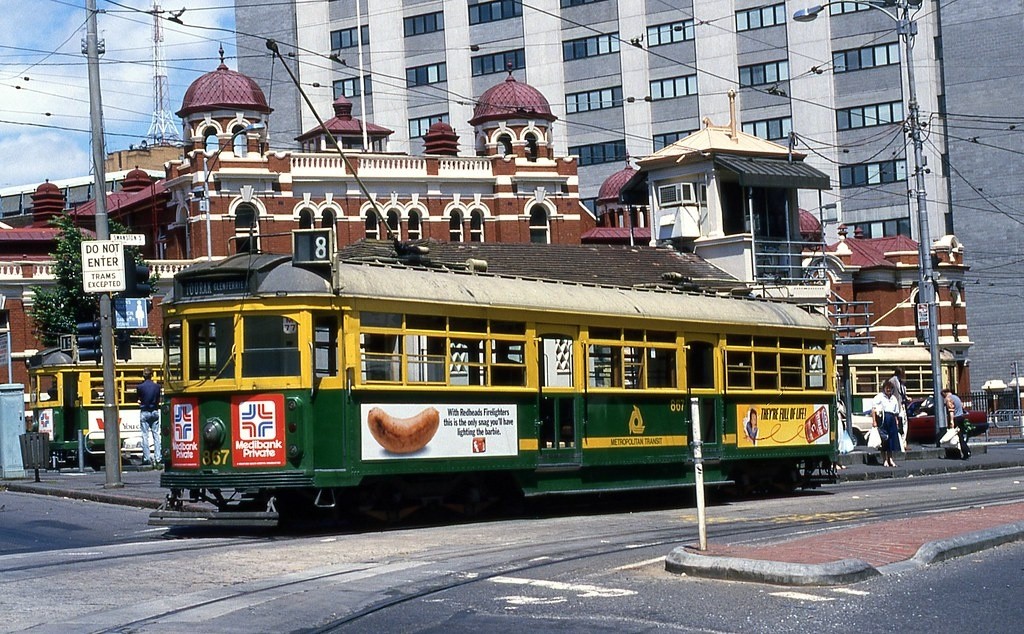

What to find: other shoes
[841,465,847,468]
[883,460,889,468]
[139,459,151,466]
[889,460,896,467]
[961,451,971,460]
[831,465,841,469]
[904,447,911,450]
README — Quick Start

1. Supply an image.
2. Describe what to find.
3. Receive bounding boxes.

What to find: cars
[848,397,988,447]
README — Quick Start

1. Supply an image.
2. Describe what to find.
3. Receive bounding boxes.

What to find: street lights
[792,1,949,448]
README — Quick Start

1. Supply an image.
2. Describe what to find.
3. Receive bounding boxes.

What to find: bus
[148,247,842,525]
[22,332,219,467]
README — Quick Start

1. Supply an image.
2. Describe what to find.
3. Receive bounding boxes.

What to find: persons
[940,389,971,460]
[870,381,900,467]
[136,366,162,463]
[888,366,913,449]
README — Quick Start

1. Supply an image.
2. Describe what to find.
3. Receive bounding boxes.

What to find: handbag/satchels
[863,427,889,450]
[939,426,960,448]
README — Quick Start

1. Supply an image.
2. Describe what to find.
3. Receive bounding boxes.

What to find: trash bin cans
[19,433,49,469]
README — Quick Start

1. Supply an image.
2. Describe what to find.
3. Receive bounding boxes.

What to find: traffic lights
[124,252,154,317]
[75,321,102,363]
